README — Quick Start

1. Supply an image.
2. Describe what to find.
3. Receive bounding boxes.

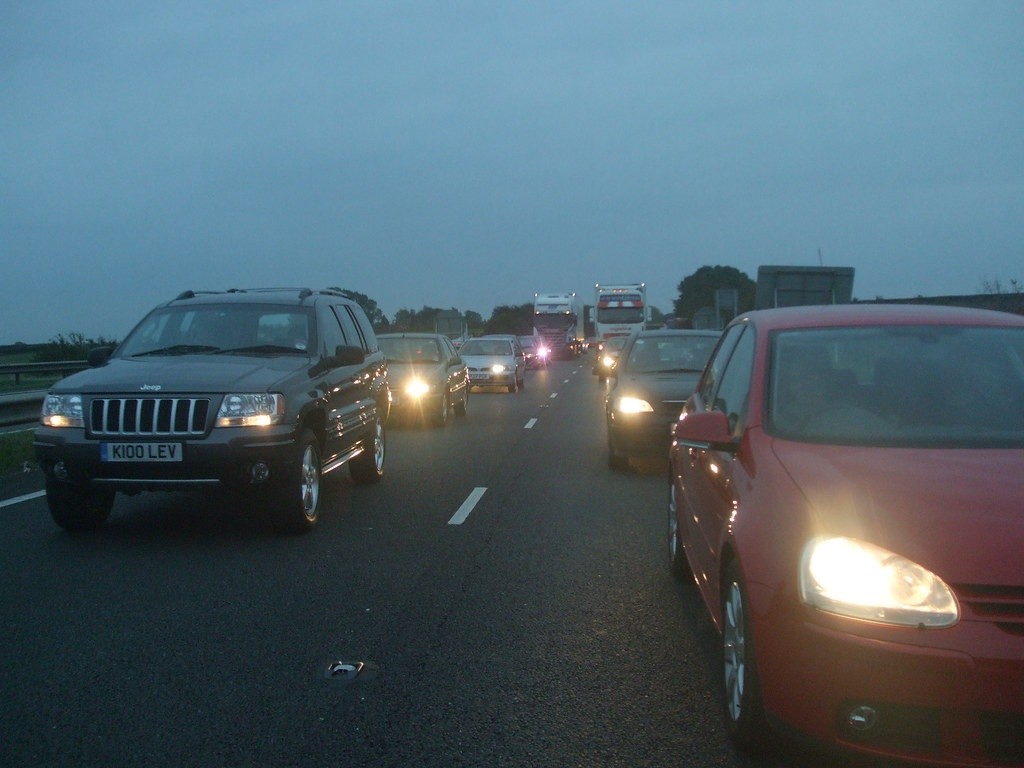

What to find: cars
[372,332,471,425]
[516,336,552,371]
[599,325,728,471]
[596,334,629,382]
[448,335,526,396]
[672,300,1024,762]
[451,339,464,349]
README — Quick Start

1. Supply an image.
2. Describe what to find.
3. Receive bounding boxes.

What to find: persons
[778,339,848,435]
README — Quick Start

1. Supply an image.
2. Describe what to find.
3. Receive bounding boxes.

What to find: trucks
[533,293,586,360]
[593,281,649,348]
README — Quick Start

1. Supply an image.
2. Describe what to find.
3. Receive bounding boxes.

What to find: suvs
[24,285,393,532]
